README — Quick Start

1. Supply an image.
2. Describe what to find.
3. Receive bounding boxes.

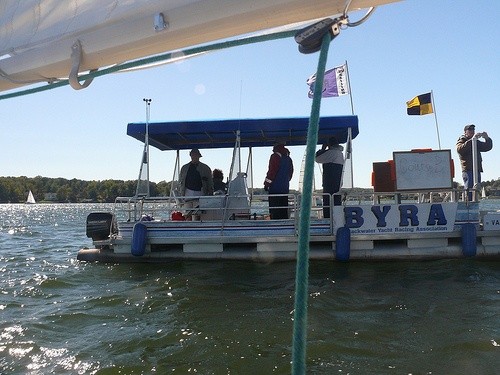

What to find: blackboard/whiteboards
[393,148,454,192]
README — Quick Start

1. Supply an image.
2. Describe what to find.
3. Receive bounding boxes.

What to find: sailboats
[25,190,37,204]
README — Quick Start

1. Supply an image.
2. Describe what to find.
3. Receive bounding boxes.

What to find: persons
[315,134,345,217]
[263,139,294,219]
[456,124,494,200]
[177,147,230,214]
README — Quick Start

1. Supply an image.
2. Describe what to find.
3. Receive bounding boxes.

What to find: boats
[78,98,500,263]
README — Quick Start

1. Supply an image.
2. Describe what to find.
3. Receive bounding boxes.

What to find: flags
[406,92,434,115]
[305,63,349,99]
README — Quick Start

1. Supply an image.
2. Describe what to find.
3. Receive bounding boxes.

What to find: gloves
[263,185,272,192]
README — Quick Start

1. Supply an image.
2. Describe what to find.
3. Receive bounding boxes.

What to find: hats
[189,146,202,156]
[464,124,475,132]
